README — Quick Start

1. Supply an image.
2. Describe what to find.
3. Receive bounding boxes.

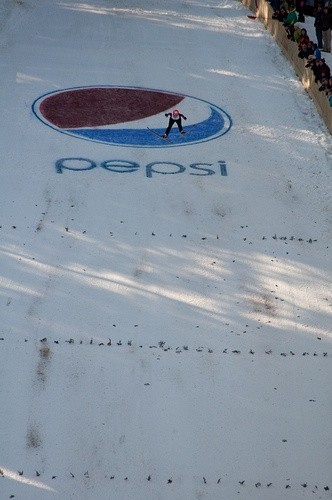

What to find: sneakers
[163,134,168,138]
[180,130,187,135]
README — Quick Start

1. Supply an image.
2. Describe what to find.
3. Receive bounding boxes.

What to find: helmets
[173,110,179,116]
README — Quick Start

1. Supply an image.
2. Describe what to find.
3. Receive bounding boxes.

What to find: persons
[163,110,187,138]
[266,0,332,106]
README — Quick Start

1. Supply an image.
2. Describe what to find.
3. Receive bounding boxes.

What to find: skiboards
[146,124,196,144]
